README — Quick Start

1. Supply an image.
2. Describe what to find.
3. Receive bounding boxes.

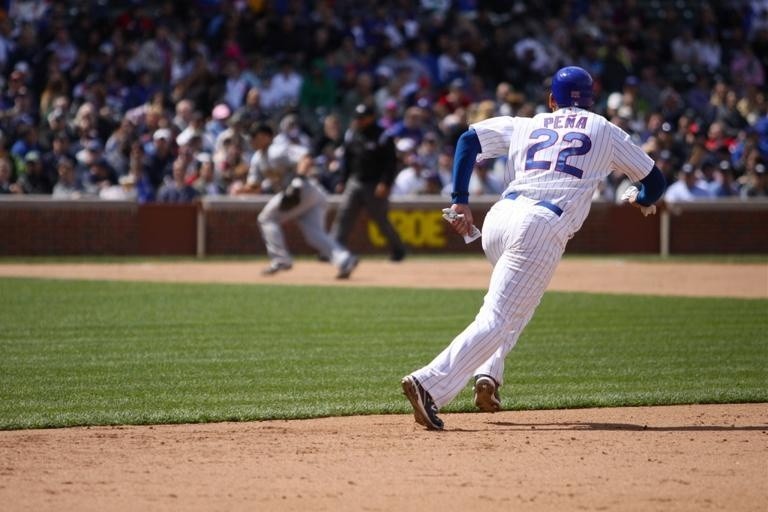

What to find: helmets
[550,64,597,109]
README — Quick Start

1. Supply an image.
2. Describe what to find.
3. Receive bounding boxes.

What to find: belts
[504,191,564,217]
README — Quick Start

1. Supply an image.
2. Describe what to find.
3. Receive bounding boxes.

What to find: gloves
[442,207,482,245]
[620,184,658,218]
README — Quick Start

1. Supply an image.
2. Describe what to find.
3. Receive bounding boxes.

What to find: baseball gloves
[280,189,302,211]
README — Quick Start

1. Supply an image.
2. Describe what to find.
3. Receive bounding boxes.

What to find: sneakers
[400,374,445,430]
[264,258,292,274]
[475,374,499,412]
[336,253,358,279]
[391,246,405,260]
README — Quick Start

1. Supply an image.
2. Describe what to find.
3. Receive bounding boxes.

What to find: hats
[607,75,641,121]
[153,98,276,147]
[346,98,374,120]
[395,78,475,155]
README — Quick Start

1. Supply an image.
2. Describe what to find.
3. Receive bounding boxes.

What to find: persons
[401,66,665,430]
[0,0,768,279]
